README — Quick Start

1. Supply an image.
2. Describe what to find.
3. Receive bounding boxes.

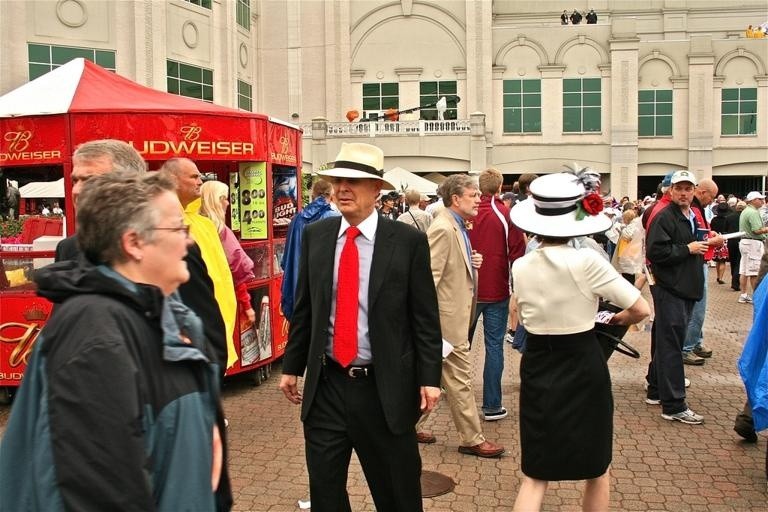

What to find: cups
[695,228,709,242]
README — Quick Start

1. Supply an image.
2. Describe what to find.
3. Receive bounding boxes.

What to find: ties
[332,225,359,366]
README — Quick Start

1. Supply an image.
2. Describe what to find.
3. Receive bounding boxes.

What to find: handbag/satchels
[593,299,640,363]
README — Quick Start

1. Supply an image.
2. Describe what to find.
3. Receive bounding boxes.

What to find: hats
[746,190,766,202]
[712,202,734,216]
[314,141,397,190]
[509,172,613,238]
[420,193,432,202]
[381,195,397,201]
[737,201,746,207]
[670,169,698,187]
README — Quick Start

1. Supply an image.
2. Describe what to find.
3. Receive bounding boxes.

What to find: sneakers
[645,394,662,406]
[716,278,727,285]
[738,294,753,304]
[506,330,515,343]
[484,406,507,422]
[693,345,712,358]
[733,415,758,442]
[661,408,704,424]
[680,350,705,366]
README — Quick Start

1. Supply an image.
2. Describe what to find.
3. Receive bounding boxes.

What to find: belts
[328,362,371,380]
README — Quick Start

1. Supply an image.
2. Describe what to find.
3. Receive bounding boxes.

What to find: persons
[278,140,441,511]
[414,168,540,460]
[639,169,768,442]
[0,139,257,512]
[510,174,651,512]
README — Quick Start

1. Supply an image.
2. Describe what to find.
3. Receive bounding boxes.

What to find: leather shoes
[458,440,506,457]
[415,431,437,444]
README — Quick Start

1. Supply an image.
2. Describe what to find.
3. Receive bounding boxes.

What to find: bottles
[239,302,261,367]
[257,296,270,349]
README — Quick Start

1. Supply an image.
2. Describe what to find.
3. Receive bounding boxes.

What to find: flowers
[571,193,603,224]
